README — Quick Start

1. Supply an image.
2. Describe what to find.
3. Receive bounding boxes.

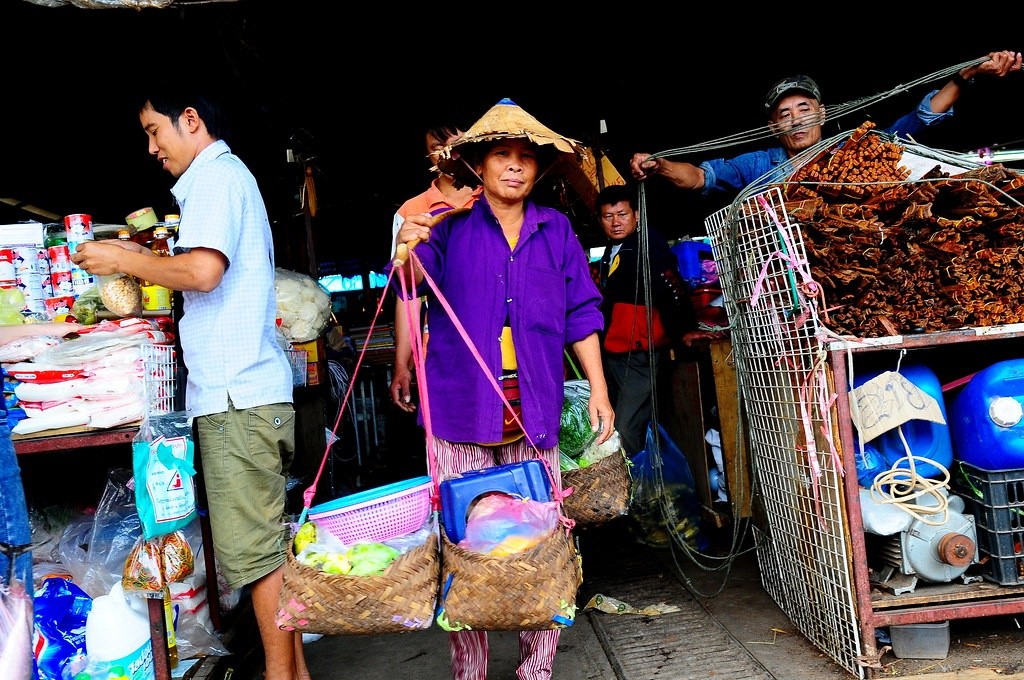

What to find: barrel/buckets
[949,358,1024,469]
[846,366,955,481]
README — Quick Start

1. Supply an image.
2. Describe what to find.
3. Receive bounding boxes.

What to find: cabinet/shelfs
[12,415,233,679]
[658,343,754,527]
[775,319,1024,679]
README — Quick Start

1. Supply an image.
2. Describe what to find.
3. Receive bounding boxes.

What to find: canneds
[0,214,101,324]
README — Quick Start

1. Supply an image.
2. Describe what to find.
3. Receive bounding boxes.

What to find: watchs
[953,73,974,88]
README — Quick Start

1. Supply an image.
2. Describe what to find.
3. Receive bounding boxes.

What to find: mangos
[295,521,397,577]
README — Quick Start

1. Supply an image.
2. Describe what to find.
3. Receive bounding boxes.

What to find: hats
[427,97,578,192]
[763,75,822,121]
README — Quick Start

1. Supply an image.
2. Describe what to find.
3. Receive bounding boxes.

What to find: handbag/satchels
[631,423,709,551]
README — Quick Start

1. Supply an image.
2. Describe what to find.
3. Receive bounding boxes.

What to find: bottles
[118,215,180,316]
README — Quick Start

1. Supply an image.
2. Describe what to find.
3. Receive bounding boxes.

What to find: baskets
[277,538,440,635]
[307,475,432,514]
[309,481,436,544]
[436,524,580,630]
[561,448,630,535]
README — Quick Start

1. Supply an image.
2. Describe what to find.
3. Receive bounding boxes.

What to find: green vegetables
[559,379,598,471]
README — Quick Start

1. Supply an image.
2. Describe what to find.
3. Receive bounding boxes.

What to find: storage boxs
[284,337,327,385]
[950,459,1024,587]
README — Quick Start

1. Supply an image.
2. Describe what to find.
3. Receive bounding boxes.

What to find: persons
[389,106,484,413]
[629,49,1022,194]
[384,95,616,680]
[70,80,311,680]
[594,185,692,459]
